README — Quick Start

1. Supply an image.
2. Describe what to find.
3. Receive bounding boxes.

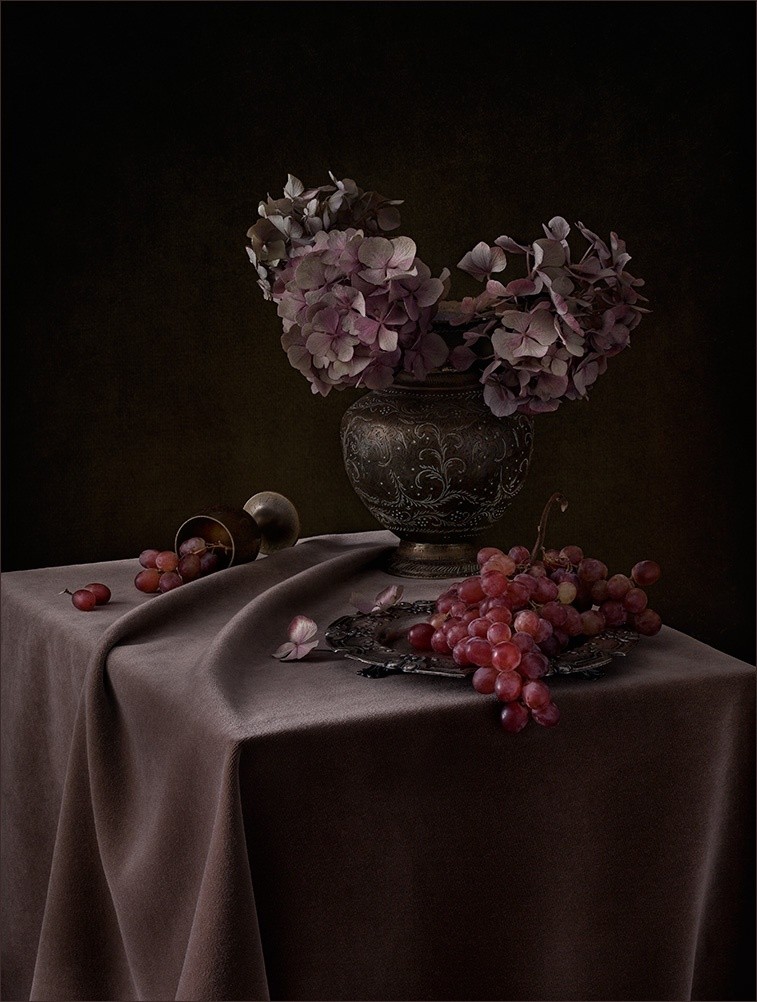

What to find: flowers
[220,175,650,423]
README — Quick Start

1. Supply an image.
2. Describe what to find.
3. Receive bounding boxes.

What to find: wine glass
[175,491,301,584]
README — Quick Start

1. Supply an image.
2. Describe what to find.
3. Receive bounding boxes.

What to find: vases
[332,375,535,583]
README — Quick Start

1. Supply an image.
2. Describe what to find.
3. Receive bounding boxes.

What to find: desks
[0,527,757,1002]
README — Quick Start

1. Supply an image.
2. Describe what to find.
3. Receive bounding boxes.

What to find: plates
[325,599,641,684]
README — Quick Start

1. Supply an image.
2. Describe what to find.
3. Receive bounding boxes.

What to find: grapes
[403,546,664,734]
[70,583,111,610]
[134,538,220,595]
